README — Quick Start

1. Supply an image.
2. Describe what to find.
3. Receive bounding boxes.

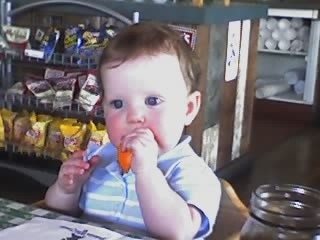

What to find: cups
[252,61,307,101]
[258,16,310,54]
[239,183,320,240]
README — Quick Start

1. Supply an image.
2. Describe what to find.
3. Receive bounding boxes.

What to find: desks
[11,0,268,172]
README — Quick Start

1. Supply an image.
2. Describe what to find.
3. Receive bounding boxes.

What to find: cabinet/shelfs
[254,7,320,122]
[0,0,139,161]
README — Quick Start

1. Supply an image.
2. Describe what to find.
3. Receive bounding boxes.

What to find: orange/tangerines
[118,143,132,174]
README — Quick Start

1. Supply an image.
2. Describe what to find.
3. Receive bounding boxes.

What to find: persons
[45,21,222,240]
